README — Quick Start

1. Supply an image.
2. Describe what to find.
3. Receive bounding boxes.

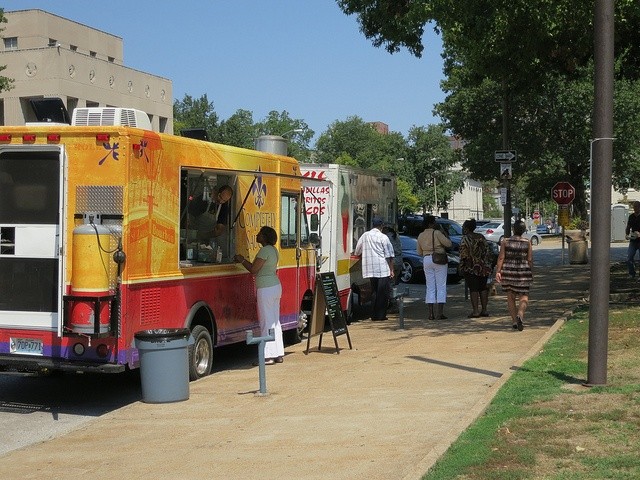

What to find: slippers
[479,312,488,317]
[468,313,480,318]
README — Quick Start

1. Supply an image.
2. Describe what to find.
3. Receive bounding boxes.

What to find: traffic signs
[493,149,517,163]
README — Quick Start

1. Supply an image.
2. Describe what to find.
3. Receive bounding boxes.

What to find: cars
[400,234,461,285]
[473,220,490,233]
[537,225,549,234]
[474,220,543,245]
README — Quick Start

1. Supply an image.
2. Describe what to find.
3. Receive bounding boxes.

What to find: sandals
[513,324,517,329]
[517,316,523,331]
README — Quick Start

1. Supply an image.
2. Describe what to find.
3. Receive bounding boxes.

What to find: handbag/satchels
[486,275,494,288]
[389,294,404,314]
[432,252,448,265]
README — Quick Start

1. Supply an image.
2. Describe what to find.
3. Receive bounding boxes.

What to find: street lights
[280,129,303,138]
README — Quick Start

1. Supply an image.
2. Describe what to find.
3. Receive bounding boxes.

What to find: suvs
[399,215,499,268]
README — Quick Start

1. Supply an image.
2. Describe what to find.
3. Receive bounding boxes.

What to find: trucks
[0,98,325,384]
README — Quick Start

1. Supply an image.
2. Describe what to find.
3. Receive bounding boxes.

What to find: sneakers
[252,359,274,365]
[275,357,283,363]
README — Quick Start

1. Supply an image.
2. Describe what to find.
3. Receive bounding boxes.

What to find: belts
[425,254,433,256]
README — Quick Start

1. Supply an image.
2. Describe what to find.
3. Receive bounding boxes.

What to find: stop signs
[550,182,576,206]
[532,212,540,220]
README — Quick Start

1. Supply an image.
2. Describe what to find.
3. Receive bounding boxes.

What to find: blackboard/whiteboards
[319,271,352,353]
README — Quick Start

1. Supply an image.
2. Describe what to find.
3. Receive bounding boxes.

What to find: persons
[382,226,403,314]
[495,220,535,332]
[182,184,234,248]
[235,226,285,366]
[458,218,496,318]
[354,214,395,321]
[417,215,453,320]
[624,201,640,279]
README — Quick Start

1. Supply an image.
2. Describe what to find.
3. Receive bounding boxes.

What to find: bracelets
[242,259,247,264]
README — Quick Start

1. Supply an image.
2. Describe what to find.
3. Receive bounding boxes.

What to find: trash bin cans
[134,328,190,404]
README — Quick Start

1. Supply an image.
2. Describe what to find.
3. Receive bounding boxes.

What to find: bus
[300,162,400,323]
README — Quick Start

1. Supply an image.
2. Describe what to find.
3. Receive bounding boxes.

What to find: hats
[372,216,385,225]
